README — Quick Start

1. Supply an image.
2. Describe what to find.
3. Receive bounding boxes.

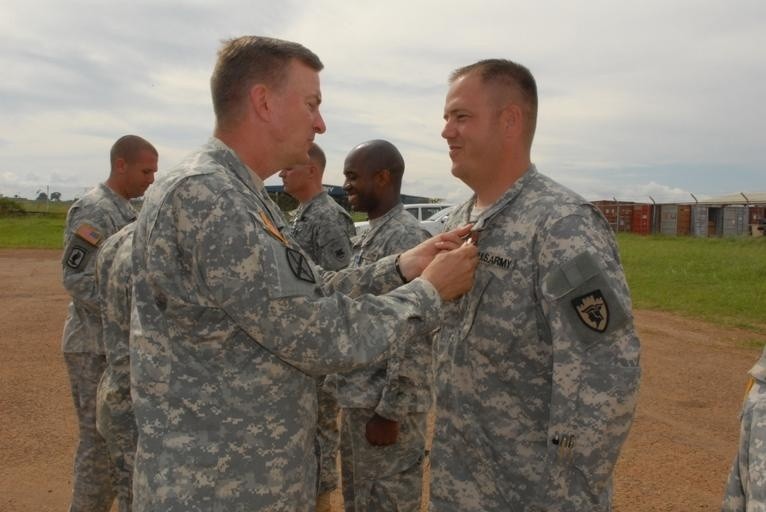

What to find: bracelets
[393,258,410,285]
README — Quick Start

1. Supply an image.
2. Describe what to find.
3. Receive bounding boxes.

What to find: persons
[129,33,480,510]
[426,55,643,512]
[341,135,436,509]
[58,132,162,512]
[717,343,766,511]
[91,212,141,512]
[276,143,357,495]
